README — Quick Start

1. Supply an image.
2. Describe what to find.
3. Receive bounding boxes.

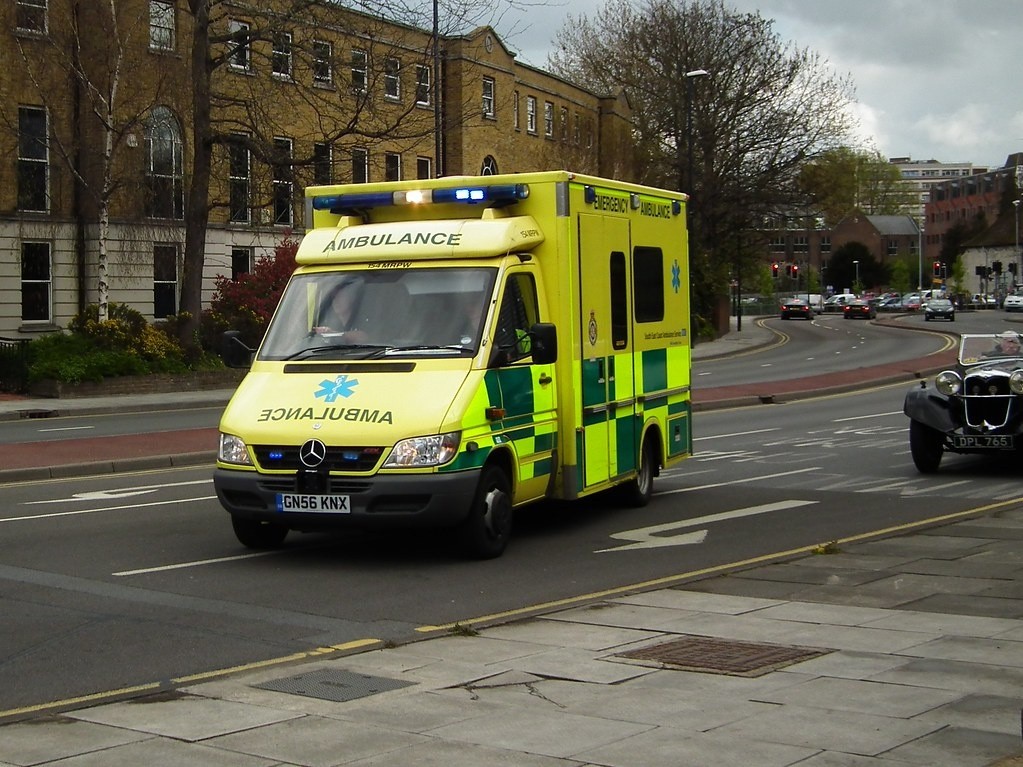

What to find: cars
[780,284,1023,322]
[903,331,1023,473]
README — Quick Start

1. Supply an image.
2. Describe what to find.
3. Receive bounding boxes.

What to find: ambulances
[213,170,694,559]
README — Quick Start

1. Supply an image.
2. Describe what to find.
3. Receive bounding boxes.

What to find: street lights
[853,261,859,286]
[686,69,709,349]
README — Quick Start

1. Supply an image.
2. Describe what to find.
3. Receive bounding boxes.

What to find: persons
[826,293,831,299]
[884,292,894,299]
[1008,280,1016,293]
[982,331,1020,358]
[429,291,516,360]
[995,294,1004,309]
[948,292,954,304]
[313,282,372,343]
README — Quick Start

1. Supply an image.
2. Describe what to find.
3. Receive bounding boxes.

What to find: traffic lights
[976,262,1017,278]
[933,261,946,278]
[772,264,779,278]
[791,265,798,281]
